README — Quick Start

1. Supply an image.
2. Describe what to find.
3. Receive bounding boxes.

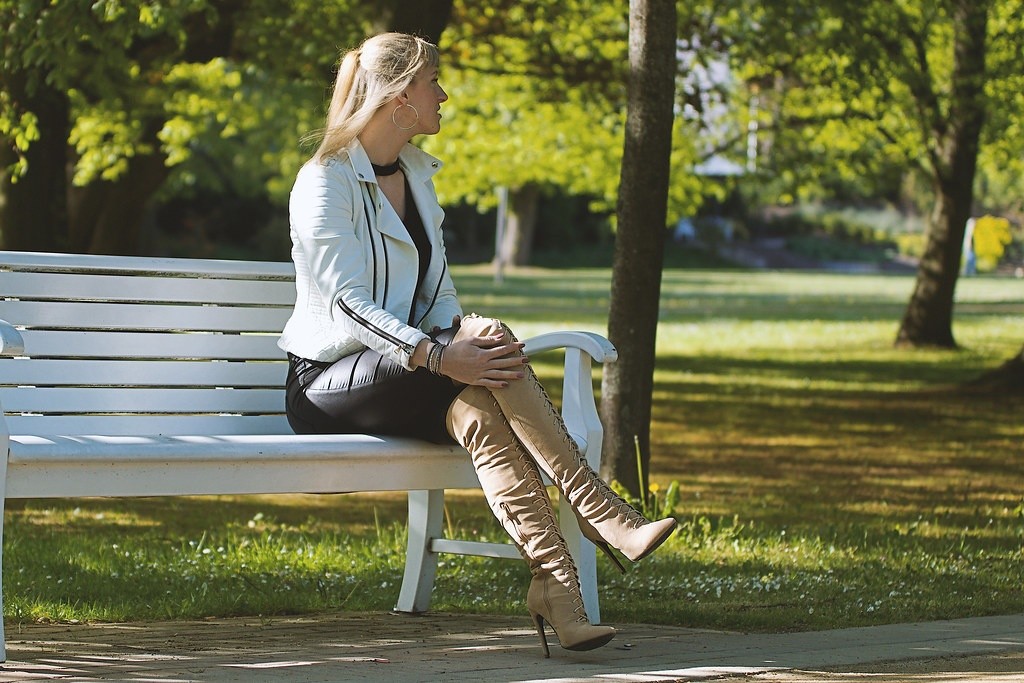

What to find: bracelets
[426,344,446,378]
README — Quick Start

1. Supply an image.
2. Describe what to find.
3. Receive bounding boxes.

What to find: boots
[444,384,618,660]
[451,311,679,575]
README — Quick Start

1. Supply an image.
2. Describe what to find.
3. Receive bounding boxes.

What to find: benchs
[0,248,620,627]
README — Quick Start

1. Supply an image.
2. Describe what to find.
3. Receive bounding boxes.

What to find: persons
[277,32,679,653]
